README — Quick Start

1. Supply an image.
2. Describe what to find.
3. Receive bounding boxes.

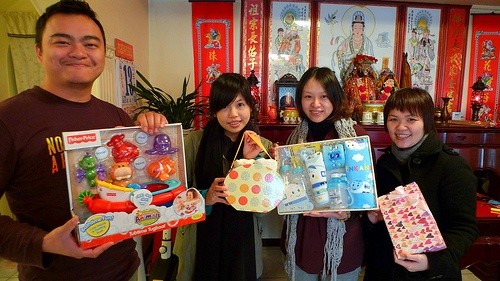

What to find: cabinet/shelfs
[257,124,500,174]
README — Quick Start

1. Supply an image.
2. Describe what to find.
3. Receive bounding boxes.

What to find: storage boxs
[274,135,380,215]
[61,123,206,250]
[379,181,447,259]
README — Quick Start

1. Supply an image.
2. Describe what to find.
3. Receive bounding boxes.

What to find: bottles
[280,160,312,201]
[433,107,442,123]
[328,174,353,210]
[269,105,278,124]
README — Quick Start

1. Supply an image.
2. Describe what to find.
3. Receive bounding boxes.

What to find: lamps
[469,76,488,124]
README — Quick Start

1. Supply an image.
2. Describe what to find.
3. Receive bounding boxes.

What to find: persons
[0,0,169,281]
[363,86,478,281]
[344,61,396,113]
[173,72,274,281]
[268,67,369,281]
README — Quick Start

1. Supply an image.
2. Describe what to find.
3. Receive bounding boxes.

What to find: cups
[362,112,372,125]
[377,112,385,125]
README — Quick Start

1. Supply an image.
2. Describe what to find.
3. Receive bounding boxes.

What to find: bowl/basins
[95,179,134,203]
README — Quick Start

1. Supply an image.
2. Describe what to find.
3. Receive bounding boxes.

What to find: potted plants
[127,69,210,135]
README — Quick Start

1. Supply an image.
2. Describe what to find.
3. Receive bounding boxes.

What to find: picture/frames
[273,74,300,121]
[261,0,315,120]
[400,2,445,111]
[313,0,402,97]
